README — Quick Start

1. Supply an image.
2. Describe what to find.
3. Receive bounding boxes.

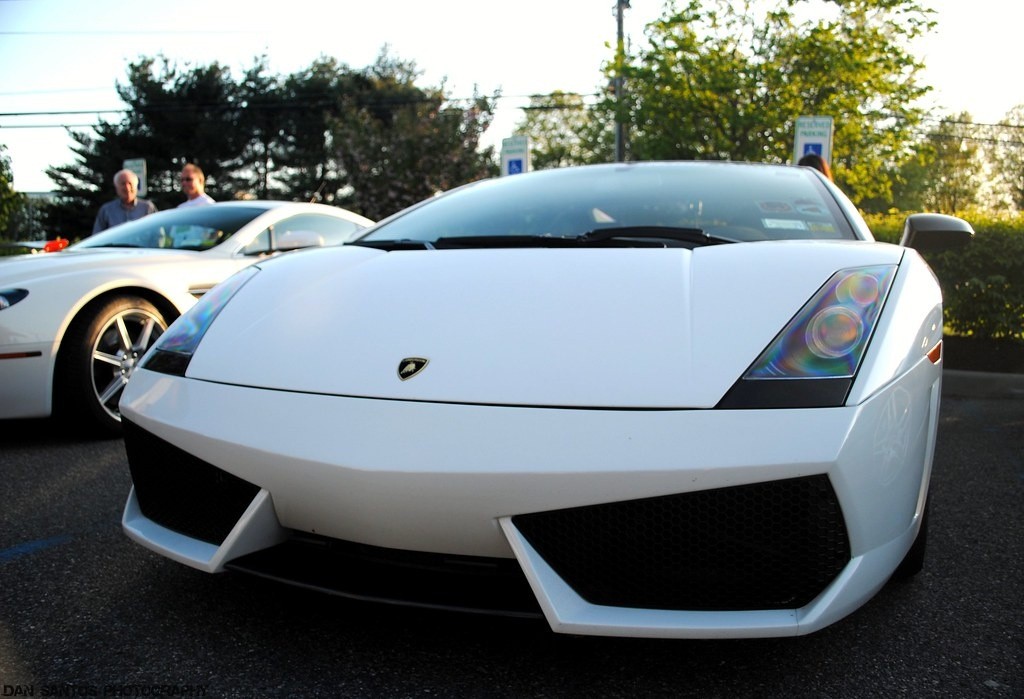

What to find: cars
[1,199,376,443]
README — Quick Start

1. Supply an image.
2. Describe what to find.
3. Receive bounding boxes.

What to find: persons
[92,169,157,235]
[798,155,831,177]
[177,164,216,206]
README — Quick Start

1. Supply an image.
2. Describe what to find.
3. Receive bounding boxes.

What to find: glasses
[179,177,200,182]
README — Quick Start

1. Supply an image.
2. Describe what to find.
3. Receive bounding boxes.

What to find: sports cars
[117,159,974,641]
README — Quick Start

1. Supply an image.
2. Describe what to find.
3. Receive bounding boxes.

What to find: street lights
[612,0,632,160]
